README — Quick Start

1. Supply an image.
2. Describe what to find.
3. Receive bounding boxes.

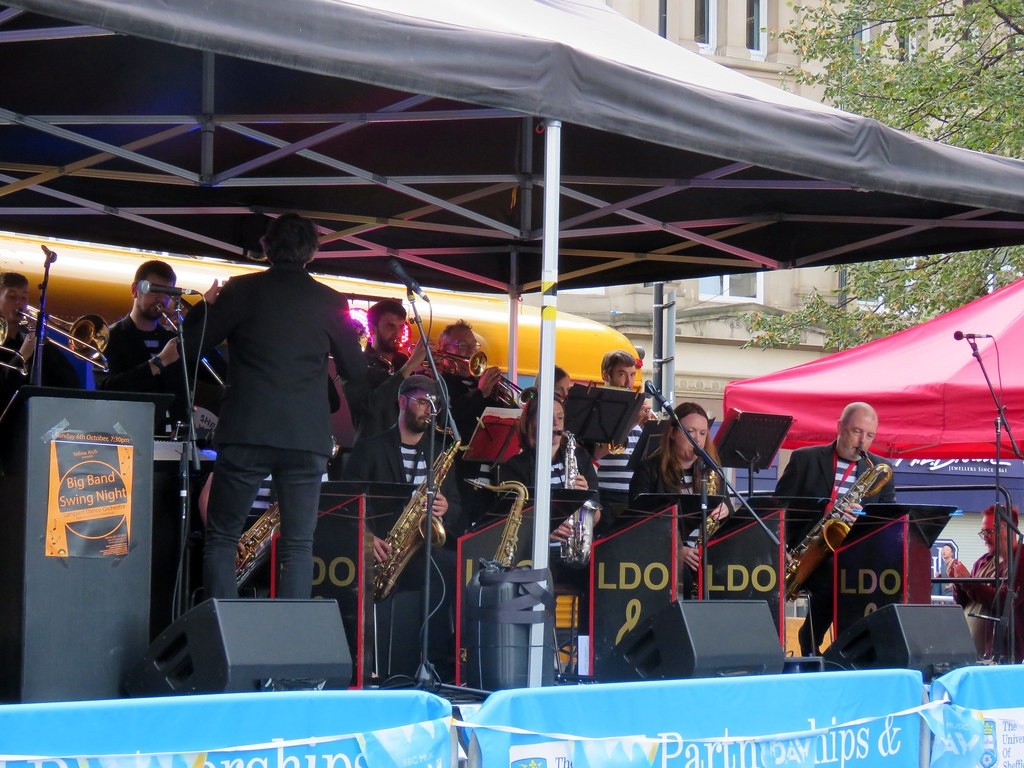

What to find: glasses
[978,525,1007,539]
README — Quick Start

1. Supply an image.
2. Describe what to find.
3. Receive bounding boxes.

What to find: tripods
[378,280,494,697]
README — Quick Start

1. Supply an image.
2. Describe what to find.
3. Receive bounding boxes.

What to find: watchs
[152,356,165,372]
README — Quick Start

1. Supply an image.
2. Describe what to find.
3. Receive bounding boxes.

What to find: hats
[398,375,437,394]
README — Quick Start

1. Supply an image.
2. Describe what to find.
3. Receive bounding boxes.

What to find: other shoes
[799,629,821,657]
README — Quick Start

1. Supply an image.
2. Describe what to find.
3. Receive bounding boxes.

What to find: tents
[723,278,1024,502]
[0,0,1024,692]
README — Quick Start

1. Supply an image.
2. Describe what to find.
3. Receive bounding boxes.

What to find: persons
[0,262,1024,687]
[775,401,896,657]
[182,211,376,609]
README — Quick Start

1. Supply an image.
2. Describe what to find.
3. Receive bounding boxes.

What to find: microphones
[387,259,428,300]
[643,380,653,399]
[953,331,991,340]
[138,280,198,295]
[42,245,57,263]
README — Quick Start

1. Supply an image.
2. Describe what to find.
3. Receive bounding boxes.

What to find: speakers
[132,598,352,696]
[821,603,976,683]
[596,599,785,682]
